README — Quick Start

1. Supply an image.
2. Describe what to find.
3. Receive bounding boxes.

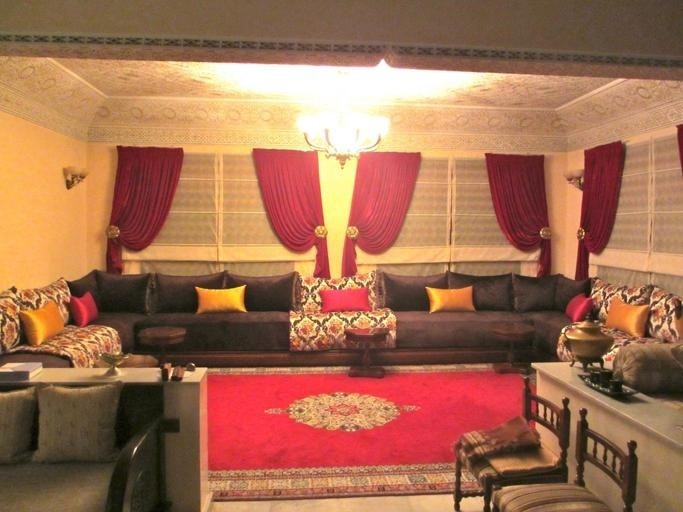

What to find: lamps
[293,104,391,164]
[64,165,88,190]
[564,170,586,190]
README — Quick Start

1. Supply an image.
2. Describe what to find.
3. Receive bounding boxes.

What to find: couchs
[97,266,536,364]
[538,274,683,364]
[0,270,133,372]
[1,385,178,511]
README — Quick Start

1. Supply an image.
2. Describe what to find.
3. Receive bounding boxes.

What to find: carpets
[208,366,543,500]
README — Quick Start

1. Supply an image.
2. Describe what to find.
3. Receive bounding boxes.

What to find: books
[0,362,43,380]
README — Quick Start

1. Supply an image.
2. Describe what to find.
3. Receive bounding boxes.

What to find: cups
[589,368,622,393]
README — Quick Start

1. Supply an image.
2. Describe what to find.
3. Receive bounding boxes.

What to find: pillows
[103,273,154,315]
[317,286,371,314]
[31,383,123,464]
[0,387,35,466]
[193,283,247,314]
[225,271,296,312]
[556,276,683,343]
[425,286,475,314]
[152,272,226,315]
[381,272,449,311]
[512,275,561,313]
[0,272,104,347]
[299,271,378,312]
[447,271,513,312]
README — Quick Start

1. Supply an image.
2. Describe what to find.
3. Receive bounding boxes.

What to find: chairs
[452,373,570,512]
[491,408,639,511]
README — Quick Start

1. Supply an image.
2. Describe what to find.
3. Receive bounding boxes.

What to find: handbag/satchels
[458,415,540,465]
